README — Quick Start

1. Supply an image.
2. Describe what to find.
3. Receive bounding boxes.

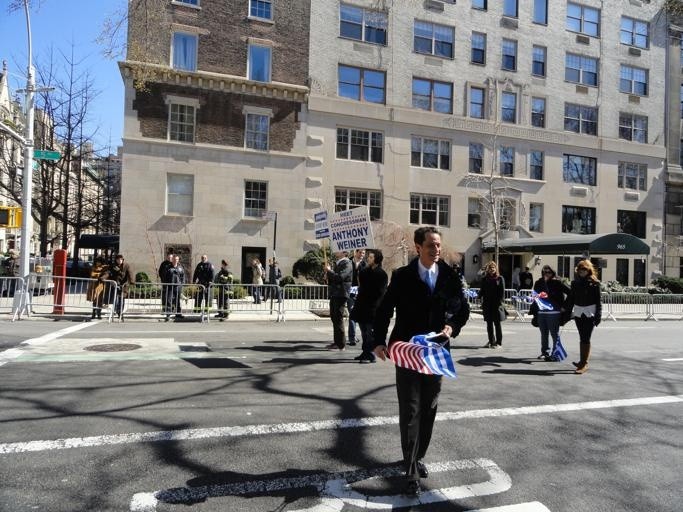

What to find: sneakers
[538,352,548,359]
[325,344,344,349]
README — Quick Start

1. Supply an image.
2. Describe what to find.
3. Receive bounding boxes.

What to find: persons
[323,248,388,364]
[269,257,281,302]
[251,259,264,302]
[474,261,505,348]
[158,253,233,321]
[4,249,20,297]
[87,257,110,318]
[513,261,601,373]
[372,228,469,499]
[453,263,467,300]
[98,255,135,320]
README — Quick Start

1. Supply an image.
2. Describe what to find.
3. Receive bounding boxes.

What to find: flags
[385,332,457,378]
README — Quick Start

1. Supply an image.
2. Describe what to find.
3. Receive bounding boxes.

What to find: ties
[424,271,434,293]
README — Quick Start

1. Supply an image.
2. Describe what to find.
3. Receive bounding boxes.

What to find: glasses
[544,271,551,274]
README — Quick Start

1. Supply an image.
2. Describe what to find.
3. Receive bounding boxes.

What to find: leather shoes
[416,459,428,478]
[214,313,222,317]
[353,351,374,361]
[406,479,420,497]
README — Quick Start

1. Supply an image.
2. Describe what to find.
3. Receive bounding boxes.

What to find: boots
[575,343,590,374]
[572,342,584,367]
